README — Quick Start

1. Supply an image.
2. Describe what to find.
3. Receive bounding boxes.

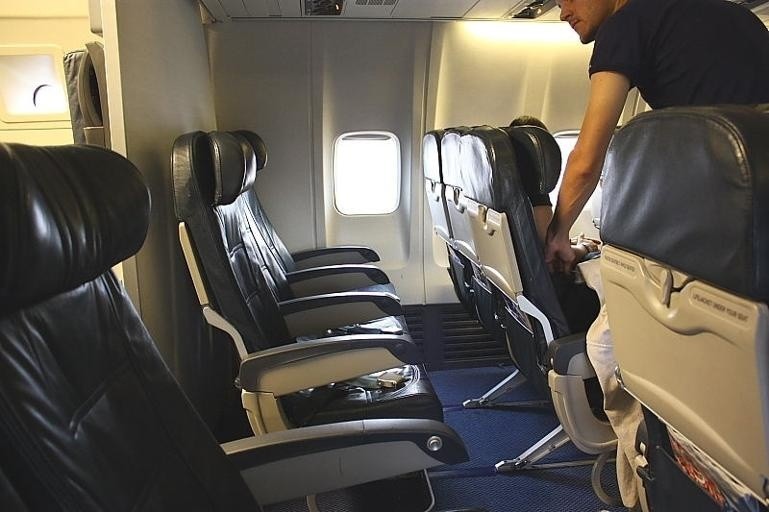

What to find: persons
[544,0,769,265]
[508,116,601,259]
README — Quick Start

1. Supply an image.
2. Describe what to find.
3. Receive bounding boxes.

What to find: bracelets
[579,243,590,253]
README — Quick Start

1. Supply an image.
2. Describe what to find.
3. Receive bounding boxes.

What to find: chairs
[0,103,472,512]
[414,102,762,510]
